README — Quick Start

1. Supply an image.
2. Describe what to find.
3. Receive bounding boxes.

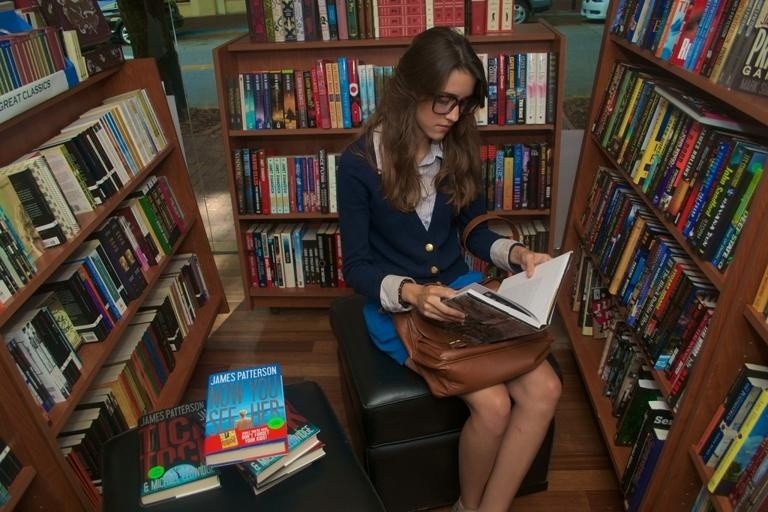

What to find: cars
[581,0,609,20]
[99,0,186,44]
[513,0,551,24]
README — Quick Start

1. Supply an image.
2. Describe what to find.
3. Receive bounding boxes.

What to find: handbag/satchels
[391,277,554,399]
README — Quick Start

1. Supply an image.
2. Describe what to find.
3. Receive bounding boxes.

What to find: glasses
[432,94,480,115]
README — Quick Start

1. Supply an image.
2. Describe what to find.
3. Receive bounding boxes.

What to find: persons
[335,26,563,512]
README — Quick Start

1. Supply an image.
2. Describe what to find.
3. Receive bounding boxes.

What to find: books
[441,249,574,345]
[240,393,322,487]
[235,440,326,496]
[203,362,290,468]
[136,399,224,505]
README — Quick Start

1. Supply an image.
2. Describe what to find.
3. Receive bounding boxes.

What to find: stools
[328,300,559,510]
[100,381,384,511]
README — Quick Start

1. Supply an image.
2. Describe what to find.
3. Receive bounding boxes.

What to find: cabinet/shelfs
[556,4,764,509]
[0,58,229,511]
[212,20,566,325]
